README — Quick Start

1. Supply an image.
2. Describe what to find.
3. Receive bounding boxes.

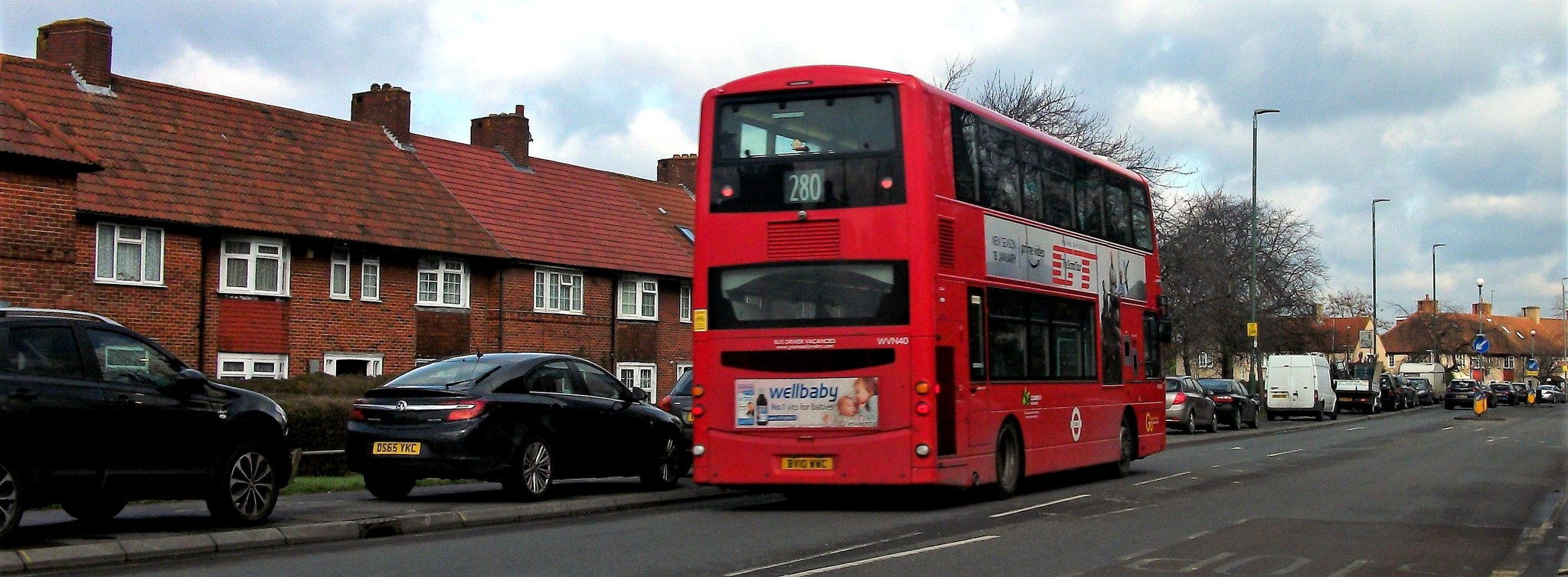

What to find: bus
[692,65,1173,503]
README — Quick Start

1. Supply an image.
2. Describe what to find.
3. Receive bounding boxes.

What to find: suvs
[0,307,303,548]
[1444,378,1486,411]
[1489,383,1520,407]
[662,367,698,478]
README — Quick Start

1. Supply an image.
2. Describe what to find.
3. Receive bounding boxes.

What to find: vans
[1264,352,1338,423]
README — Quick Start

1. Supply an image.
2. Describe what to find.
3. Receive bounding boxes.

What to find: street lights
[1432,243,1447,364]
[1476,277,1484,385]
[1372,198,1392,363]
[1530,330,1536,359]
[1247,107,1282,398]
[1562,276,1568,361]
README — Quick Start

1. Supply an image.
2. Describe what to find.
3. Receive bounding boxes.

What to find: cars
[1534,385,1567,404]
[1380,372,1433,412]
[346,353,687,502]
[1481,385,1497,408]
[1197,379,1260,432]
[1512,382,1531,405]
[1164,376,1217,435]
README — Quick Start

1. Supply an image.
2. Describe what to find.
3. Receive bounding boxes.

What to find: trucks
[1332,361,1382,416]
